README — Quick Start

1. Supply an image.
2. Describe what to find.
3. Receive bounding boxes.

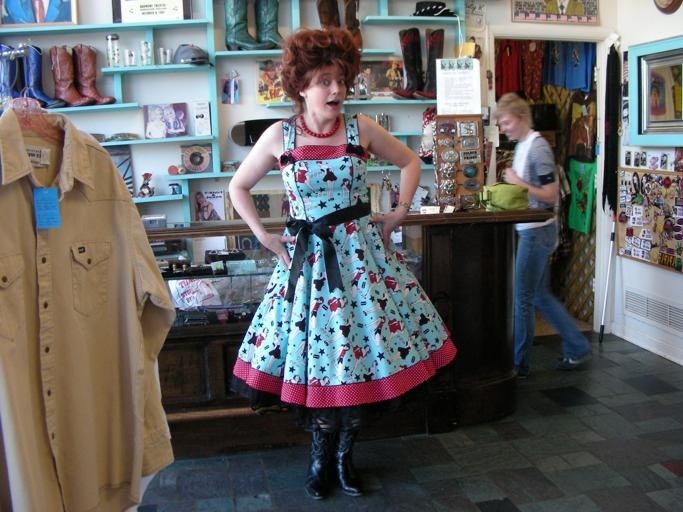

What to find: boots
[343,0,363,52]
[72,43,116,105]
[223,0,277,52]
[253,0,287,48]
[412,28,445,100]
[302,424,334,501]
[390,27,424,100]
[0,44,47,108]
[333,424,365,498]
[49,46,96,107]
[19,44,67,109]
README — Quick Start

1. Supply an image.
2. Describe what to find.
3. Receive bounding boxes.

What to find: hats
[413,1,455,17]
[173,43,209,64]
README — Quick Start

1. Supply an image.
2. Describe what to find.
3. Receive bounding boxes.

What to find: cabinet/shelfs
[0,0,466,266]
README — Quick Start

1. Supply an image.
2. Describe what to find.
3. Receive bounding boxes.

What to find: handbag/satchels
[479,180,530,212]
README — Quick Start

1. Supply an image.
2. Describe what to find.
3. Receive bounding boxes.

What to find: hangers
[1,43,65,141]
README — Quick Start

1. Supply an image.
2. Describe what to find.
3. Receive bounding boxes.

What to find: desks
[146,209,557,461]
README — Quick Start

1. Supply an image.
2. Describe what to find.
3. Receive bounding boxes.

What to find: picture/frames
[511,0,599,27]
[628,36,683,147]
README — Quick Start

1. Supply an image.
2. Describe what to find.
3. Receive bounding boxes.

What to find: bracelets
[395,200,412,210]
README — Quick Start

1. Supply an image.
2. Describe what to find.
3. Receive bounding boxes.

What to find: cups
[141,41,153,65]
[159,46,172,64]
[124,47,138,67]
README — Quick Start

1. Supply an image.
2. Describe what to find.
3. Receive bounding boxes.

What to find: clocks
[654,1,681,14]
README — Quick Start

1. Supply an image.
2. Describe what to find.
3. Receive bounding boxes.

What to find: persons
[147,108,167,138]
[163,106,186,137]
[650,86,661,111]
[470,37,481,60]
[193,191,220,222]
[670,66,681,119]
[385,61,401,89]
[522,42,543,99]
[258,59,280,93]
[227,27,458,500]
[544,0,586,15]
[626,150,673,232]
[495,93,594,380]
[347,65,372,96]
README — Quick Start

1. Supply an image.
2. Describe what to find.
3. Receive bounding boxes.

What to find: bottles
[105,33,121,67]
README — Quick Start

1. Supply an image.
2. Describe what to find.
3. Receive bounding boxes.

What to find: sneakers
[512,366,532,379]
[555,350,594,371]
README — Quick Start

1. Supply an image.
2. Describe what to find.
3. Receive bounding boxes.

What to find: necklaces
[298,112,341,138]
